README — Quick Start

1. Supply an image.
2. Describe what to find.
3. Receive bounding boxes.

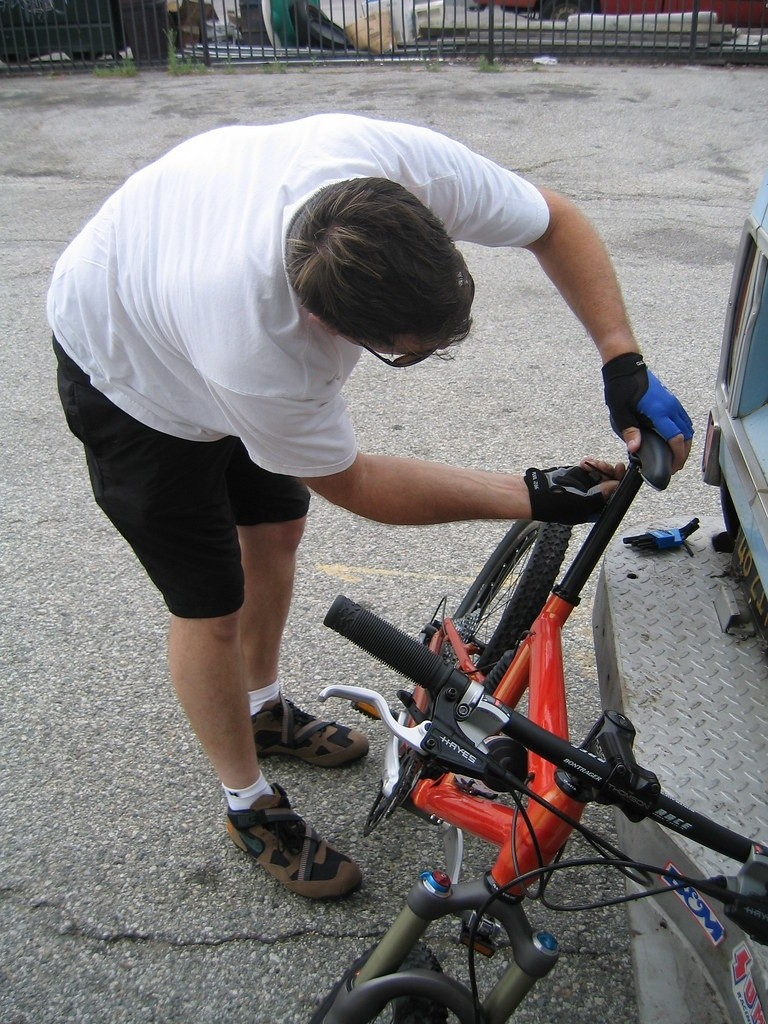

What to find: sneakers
[250,691,370,767]
[226,784,361,899]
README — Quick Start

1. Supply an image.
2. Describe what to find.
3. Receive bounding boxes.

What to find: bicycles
[305,411,768,1024]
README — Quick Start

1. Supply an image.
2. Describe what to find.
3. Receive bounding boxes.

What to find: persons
[46,111,693,904]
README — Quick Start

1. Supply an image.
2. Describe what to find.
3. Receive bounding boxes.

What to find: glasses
[339,328,441,367]
